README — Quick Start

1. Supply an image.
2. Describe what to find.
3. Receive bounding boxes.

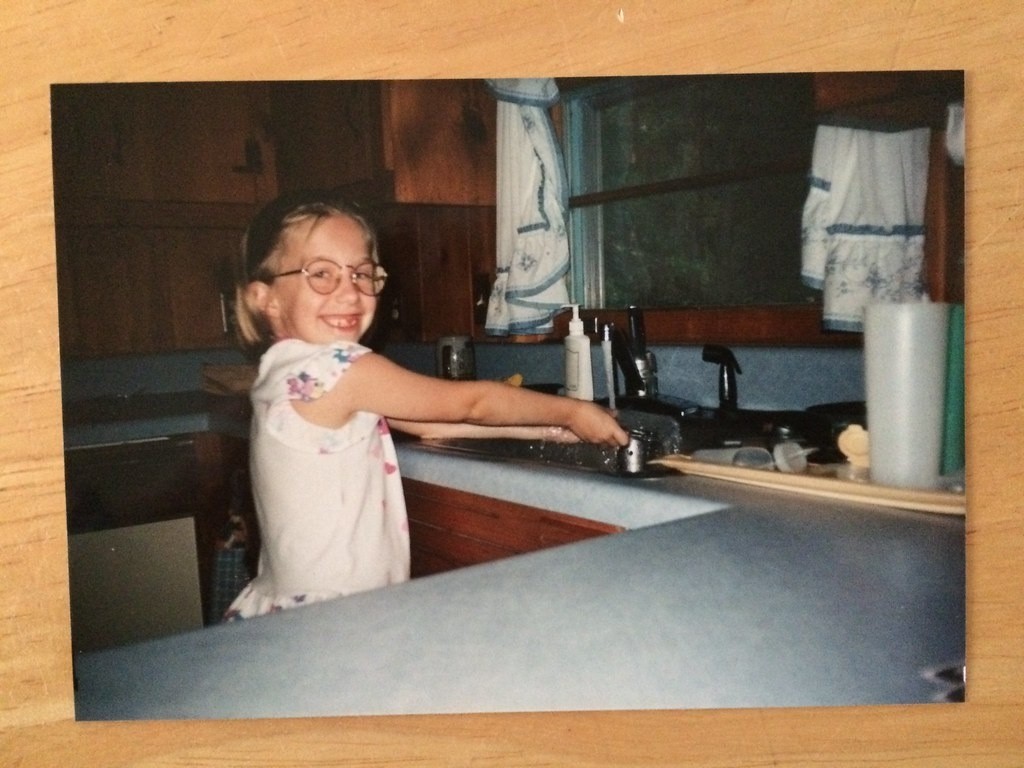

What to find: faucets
[602,421,654,474]
[603,303,660,411]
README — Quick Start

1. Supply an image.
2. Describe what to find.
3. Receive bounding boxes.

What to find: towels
[801,123,929,337]
[482,97,569,336]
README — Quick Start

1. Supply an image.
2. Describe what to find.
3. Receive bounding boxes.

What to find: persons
[219,191,631,621]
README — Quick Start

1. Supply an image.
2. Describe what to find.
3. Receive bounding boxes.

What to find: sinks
[432,411,676,468]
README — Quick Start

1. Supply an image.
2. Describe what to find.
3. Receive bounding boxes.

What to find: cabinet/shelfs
[63,433,625,610]
[50,81,498,357]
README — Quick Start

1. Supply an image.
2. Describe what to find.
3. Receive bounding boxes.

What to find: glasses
[259,259,388,295]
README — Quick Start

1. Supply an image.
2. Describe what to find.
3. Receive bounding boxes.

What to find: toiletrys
[561,302,591,406]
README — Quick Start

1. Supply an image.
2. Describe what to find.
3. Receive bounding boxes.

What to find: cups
[862,301,948,492]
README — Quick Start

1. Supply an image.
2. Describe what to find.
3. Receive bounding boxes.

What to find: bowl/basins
[578,414,682,475]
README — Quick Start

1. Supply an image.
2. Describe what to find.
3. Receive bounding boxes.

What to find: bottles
[435,335,478,381]
